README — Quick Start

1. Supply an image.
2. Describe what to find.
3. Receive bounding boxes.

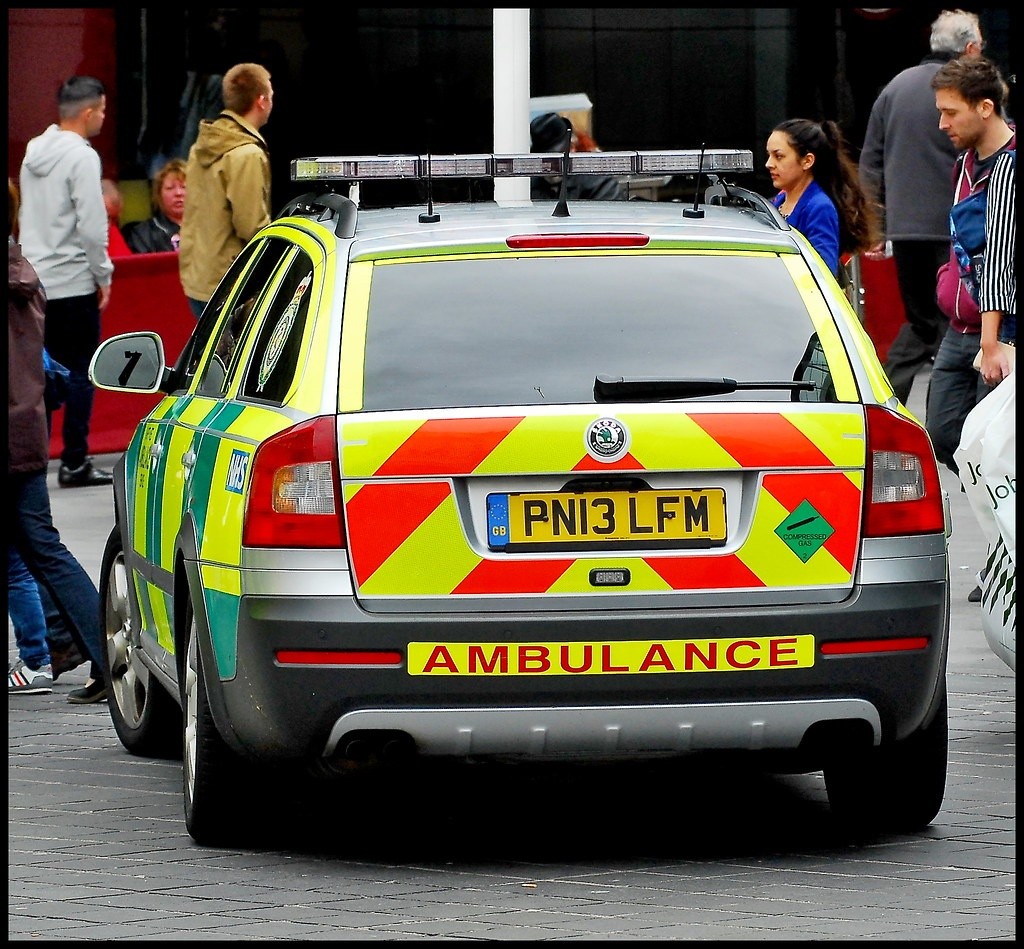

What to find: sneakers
[9,662,51,697]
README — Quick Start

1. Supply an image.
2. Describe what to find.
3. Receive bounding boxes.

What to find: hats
[532,110,571,158]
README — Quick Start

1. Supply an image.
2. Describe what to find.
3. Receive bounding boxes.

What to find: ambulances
[85,150,952,848]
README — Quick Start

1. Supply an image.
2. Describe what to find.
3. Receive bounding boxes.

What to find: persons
[923,56,1017,475]
[17,74,116,489]
[102,159,188,261]
[7,177,117,705]
[529,112,627,200]
[859,9,982,410]
[969,149,1017,601]
[766,119,871,292]
[7,544,89,695]
[181,62,272,318]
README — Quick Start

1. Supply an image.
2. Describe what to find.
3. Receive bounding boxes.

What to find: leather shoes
[58,466,114,489]
[66,680,108,702]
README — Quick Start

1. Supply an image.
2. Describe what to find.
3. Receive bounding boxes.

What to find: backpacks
[950,148,1020,346]
